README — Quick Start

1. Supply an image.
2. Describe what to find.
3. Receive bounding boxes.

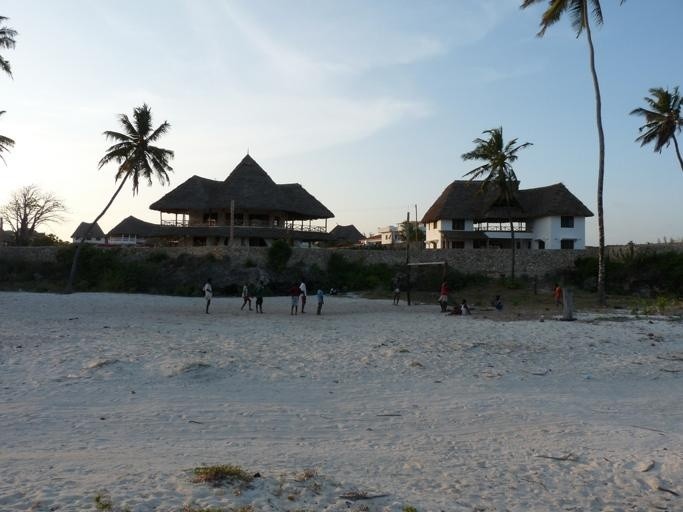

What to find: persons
[391,278,472,314]
[493,295,504,311]
[240,279,264,314]
[203,278,214,314]
[290,279,338,315]
[552,283,564,312]
